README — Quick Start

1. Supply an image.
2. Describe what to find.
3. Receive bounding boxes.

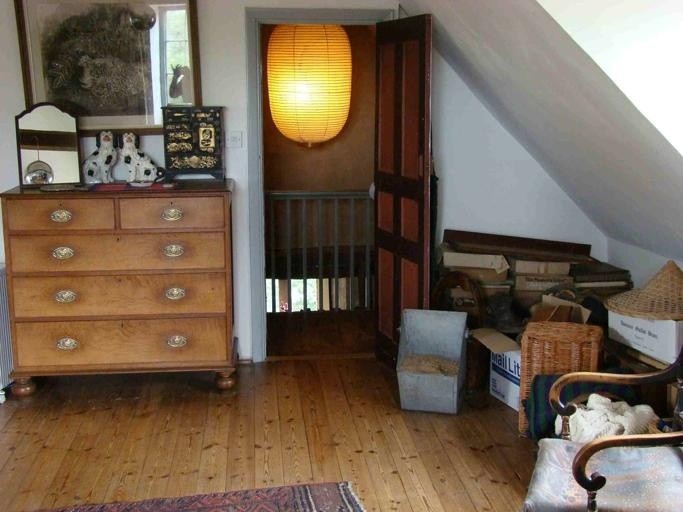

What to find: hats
[603,260,683,319]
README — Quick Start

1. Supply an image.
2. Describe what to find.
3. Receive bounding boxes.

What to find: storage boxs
[618,348,680,415]
[396,309,473,413]
[511,275,574,308]
[433,245,511,284]
[443,283,510,320]
[467,328,527,415]
[507,255,570,275]
[608,310,683,366]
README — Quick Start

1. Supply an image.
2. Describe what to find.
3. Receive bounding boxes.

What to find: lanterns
[266,22,352,148]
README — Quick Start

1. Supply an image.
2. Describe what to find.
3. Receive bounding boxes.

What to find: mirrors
[14,102,83,190]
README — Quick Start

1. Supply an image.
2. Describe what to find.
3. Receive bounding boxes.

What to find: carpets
[25,482,364,511]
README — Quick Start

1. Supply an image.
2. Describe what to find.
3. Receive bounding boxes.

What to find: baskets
[519,321,603,437]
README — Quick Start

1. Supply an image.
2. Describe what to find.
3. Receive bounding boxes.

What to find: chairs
[523,345,683,512]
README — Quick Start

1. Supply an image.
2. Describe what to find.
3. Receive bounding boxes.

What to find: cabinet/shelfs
[161,106,227,181]
[0,178,240,396]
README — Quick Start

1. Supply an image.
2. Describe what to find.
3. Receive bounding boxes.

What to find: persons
[201,129,212,147]
[169,64,192,103]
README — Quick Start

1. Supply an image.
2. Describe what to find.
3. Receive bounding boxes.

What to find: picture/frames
[14,0,203,136]
